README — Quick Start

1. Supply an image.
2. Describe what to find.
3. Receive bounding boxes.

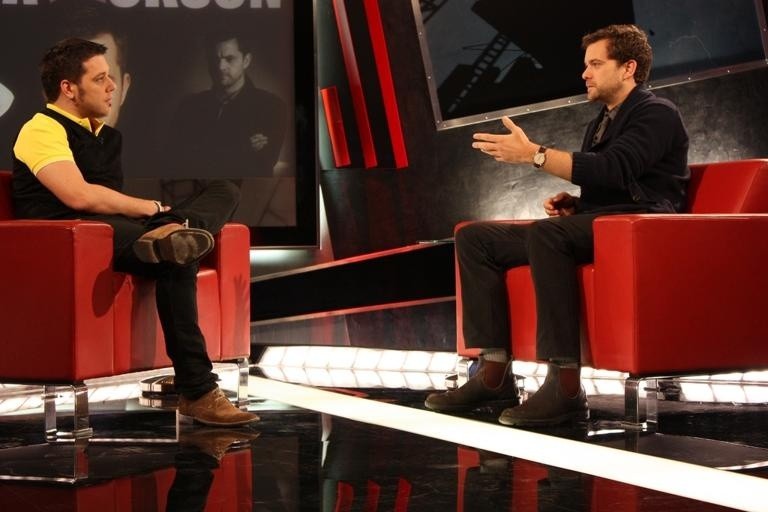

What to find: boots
[423,359,520,414]
[499,366,590,427]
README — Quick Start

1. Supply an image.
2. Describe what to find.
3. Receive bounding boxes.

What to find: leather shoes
[175,386,260,426]
[176,427,261,461]
[133,223,214,268]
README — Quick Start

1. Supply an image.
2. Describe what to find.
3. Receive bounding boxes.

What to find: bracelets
[150,199,166,215]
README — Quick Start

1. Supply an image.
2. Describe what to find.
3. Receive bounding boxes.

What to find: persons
[162,424,264,512]
[33,7,135,130]
[157,23,287,230]
[8,36,263,426]
[424,22,692,430]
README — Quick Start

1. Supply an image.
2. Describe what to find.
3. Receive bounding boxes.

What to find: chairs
[454,159,767,432]
[0,170,251,441]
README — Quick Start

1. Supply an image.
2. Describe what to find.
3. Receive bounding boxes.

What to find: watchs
[532,145,548,169]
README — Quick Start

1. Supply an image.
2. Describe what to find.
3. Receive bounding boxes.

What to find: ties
[590,105,619,148]
